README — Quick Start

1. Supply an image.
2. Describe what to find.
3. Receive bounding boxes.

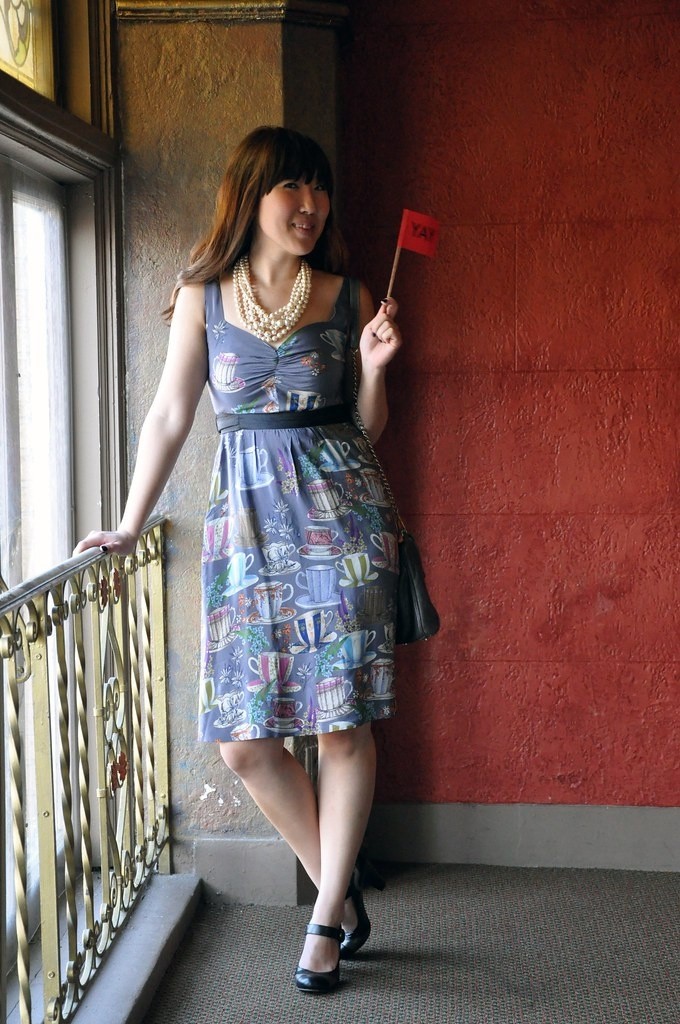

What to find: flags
[397,209,440,258]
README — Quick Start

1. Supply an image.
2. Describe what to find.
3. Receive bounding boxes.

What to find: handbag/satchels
[390,531,440,645]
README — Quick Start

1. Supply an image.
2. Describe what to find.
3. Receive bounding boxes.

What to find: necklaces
[232,256,313,342]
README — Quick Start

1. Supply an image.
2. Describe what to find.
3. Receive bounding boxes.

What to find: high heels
[340,873,373,957]
[295,922,344,995]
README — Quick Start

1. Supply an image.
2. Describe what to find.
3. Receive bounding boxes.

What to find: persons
[69,121,403,994]
